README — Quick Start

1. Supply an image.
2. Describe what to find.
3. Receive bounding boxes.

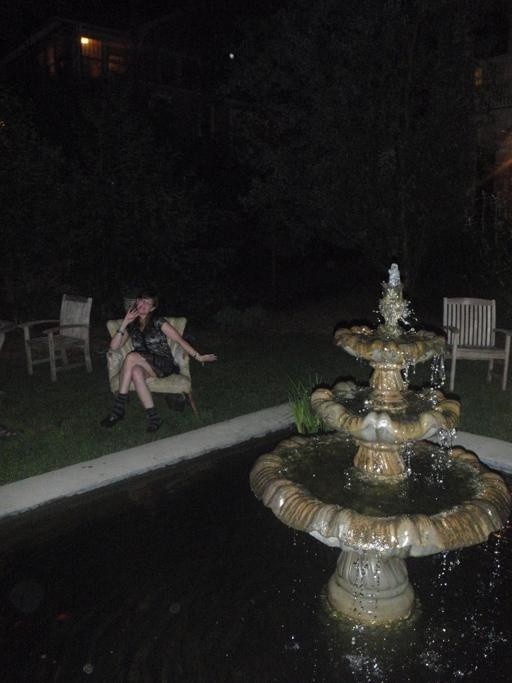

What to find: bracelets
[193,351,197,359]
[116,328,124,336]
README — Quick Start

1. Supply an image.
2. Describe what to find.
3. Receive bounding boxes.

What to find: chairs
[13,292,204,421]
[440,293,510,393]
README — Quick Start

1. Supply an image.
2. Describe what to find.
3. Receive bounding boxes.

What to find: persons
[98,290,219,434]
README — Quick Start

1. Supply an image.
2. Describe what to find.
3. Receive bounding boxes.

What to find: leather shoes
[101,410,125,428]
[148,418,163,432]
